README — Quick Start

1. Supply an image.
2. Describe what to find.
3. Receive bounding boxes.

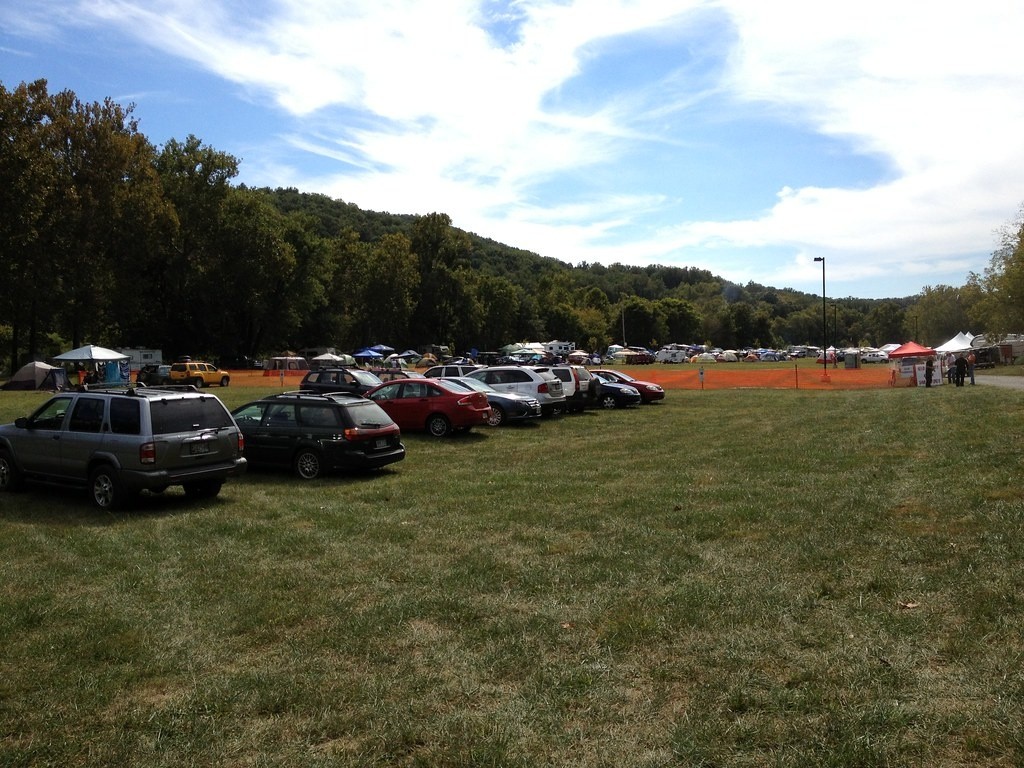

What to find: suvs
[367,369,424,382]
[0,381,247,511]
[169,362,231,389]
[228,390,405,481]
[459,366,566,418]
[534,365,601,414]
[300,365,382,397]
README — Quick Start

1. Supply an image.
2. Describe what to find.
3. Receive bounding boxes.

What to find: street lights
[830,304,836,367]
[912,316,918,342]
[814,257,826,371]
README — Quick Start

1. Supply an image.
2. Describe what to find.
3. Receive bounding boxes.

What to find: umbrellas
[826,345,839,351]
[356,344,394,351]
[312,353,344,363]
[570,351,588,356]
[352,350,383,358]
[511,349,546,355]
[53,344,130,363]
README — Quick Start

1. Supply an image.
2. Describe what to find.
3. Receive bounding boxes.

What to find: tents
[381,354,408,368]
[8,361,54,390]
[691,352,786,363]
[815,351,834,364]
[888,341,936,386]
[608,344,624,352]
[399,349,438,368]
[933,331,975,353]
[338,354,358,366]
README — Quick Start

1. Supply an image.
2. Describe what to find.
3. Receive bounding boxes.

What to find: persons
[606,349,613,355]
[945,351,976,387]
[391,359,396,370]
[587,351,600,365]
[925,356,935,388]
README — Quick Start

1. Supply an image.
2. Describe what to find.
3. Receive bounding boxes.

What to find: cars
[360,379,493,438]
[590,372,641,409]
[589,369,665,402]
[860,352,889,364]
[440,377,541,427]
[136,364,173,388]
[423,363,486,379]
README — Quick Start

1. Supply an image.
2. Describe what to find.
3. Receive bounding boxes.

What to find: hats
[945,351,952,355]
